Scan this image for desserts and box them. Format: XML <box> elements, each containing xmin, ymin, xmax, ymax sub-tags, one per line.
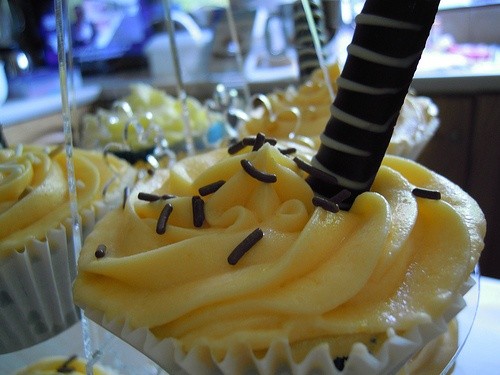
<box><xmin>79</xmin><ymin>85</ymin><xmax>210</xmax><ymax>151</ymax></box>
<box><xmin>0</xmin><ymin>143</ymin><xmax>127</xmax><ymax>261</ymax></box>
<box><xmin>72</xmin><ymin>134</ymin><xmax>486</xmax><ymax>374</ymax></box>
<box><xmin>239</xmin><ymin>58</ymin><xmax>431</xmax><ymax>143</ymax></box>
